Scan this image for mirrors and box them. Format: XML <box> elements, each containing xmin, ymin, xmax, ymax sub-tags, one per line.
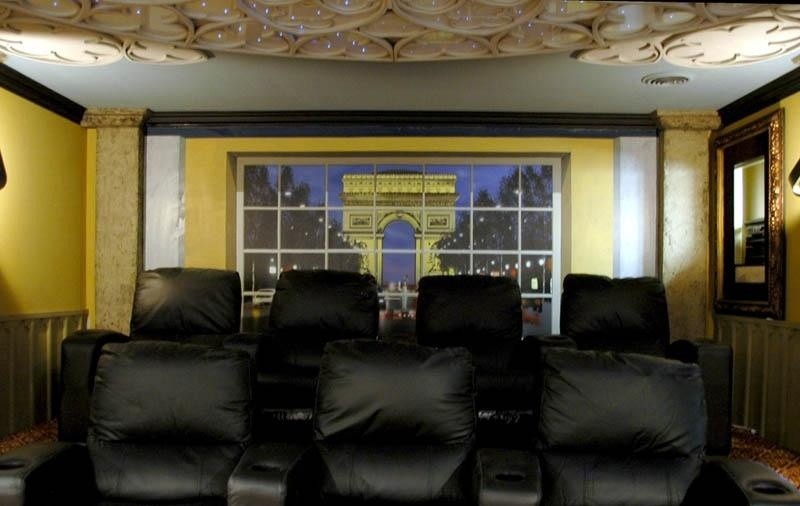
<box><xmin>709</xmin><ymin>107</ymin><xmax>786</xmax><ymax>322</ymax></box>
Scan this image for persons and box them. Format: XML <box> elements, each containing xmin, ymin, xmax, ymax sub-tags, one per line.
<box><xmin>522</xmin><ymin>298</ymin><xmax>542</xmax><ymax>313</ymax></box>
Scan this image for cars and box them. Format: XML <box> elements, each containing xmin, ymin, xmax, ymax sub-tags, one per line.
<box><xmin>253</xmin><ymin>288</ymin><xmax>276</xmax><ymax>307</ymax></box>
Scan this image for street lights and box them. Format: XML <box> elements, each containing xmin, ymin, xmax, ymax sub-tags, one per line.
<box><xmin>270</xmin><ymin>189</ymin><xmax>341</xmax><ymax>286</ymax></box>
<box><xmin>440</xmin><ymin>189</ymin><xmax>546</xmax><ymax>305</ymax></box>
